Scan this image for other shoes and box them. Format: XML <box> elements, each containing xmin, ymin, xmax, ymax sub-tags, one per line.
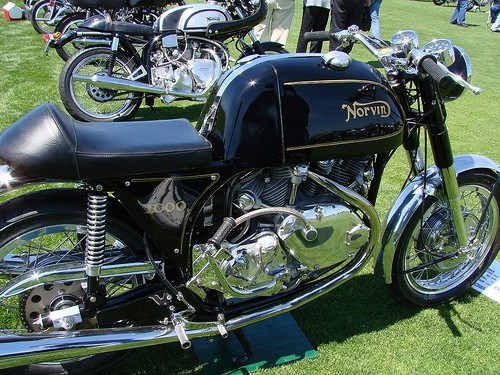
<box><xmin>459</xmin><ymin>23</ymin><xmax>469</xmax><ymax>27</ymax></box>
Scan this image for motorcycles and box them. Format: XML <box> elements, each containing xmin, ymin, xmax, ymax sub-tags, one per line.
<box><xmin>0</xmin><ymin>24</ymin><xmax>500</xmax><ymax>375</ymax></box>
<box><xmin>433</xmin><ymin>0</ymin><xmax>490</xmax><ymax>13</ymax></box>
<box><xmin>23</xmin><ymin>0</ymin><xmax>288</xmax><ymax>123</ymax></box>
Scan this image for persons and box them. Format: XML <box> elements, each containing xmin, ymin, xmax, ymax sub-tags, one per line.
<box><xmin>296</xmin><ymin>0</ymin><xmax>331</xmax><ymax>53</ymax></box>
<box><xmin>489</xmin><ymin>0</ymin><xmax>500</xmax><ymax>32</ymax></box>
<box><xmin>329</xmin><ymin>0</ymin><xmax>371</xmax><ymax>54</ymax></box>
<box><xmin>370</xmin><ymin>0</ymin><xmax>383</xmax><ymax>48</ymax></box>
<box><xmin>251</xmin><ymin>0</ymin><xmax>295</xmax><ymax>55</ymax></box>
<box><xmin>451</xmin><ymin>0</ymin><xmax>468</xmax><ymax>27</ymax></box>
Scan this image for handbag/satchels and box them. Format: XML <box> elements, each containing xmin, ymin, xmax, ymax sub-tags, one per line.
<box><xmin>361</xmin><ymin>5</ymin><xmax>372</xmax><ymax>32</ymax></box>
